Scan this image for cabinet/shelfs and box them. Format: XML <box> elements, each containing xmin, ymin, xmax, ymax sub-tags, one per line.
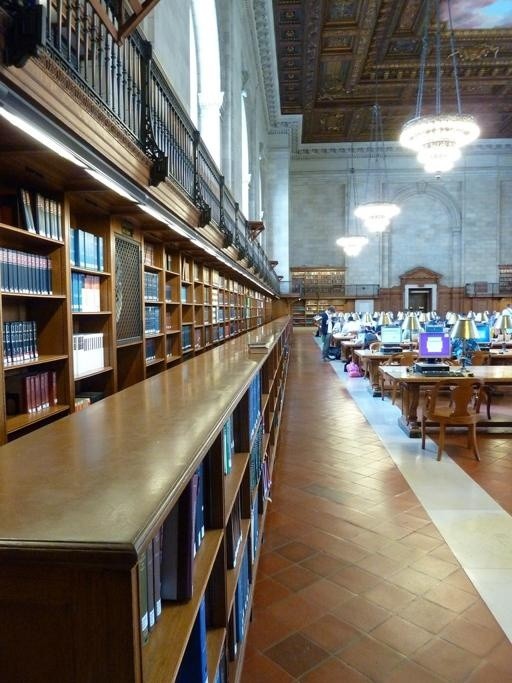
<box><xmin>1</xmin><ymin>131</ymin><xmax>292</xmax><ymax>683</ymax></box>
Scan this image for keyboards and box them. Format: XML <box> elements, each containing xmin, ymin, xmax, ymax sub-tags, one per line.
<box><xmin>383</xmin><ymin>352</ymin><xmax>397</xmax><ymax>354</ymax></box>
<box><xmin>422</xmin><ymin>371</ymin><xmax>465</xmax><ymax>377</ymax></box>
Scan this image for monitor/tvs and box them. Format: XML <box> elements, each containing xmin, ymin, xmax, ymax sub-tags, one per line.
<box><xmin>474</xmin><ymin>325</ymin><xmax>491</xmax><ymax>344</ymax></box>
<box><xmin>425</xmin><ymin>325</ymin><xmax>444</xmax><ymax>332</ymax></box>
<box><xmin>417</xmin><ymin>332</ymin><xmax>452</xmax><ymax>364</ymax></box>
<box><xmin>381</xmin><ymin>326</ymin><xmax>402</xmax><ymax>347</ymax></box>
<box><xmin>358</xmin><ymin>332</ymin><xmax>366</xmax><ymax>342</ymax></box>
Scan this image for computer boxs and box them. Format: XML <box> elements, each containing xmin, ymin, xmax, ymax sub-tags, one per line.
<box><xmin>480</xmin><ymin>347</ymin><xmax>490</xmax><ymax>352</ymax></box>
<box><xmin>380</xmin><ymin>346</ymin><xmax>402</xmax><ymax>353</ymax></box>
<box><xmin>416</xmin><ymin>362</ymin><xmax>449</xmax><ymax>374</ymax></box>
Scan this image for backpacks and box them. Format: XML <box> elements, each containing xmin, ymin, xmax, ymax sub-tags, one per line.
<box><xmin>345</xmin><ymin>361</ymin><xmax>362</xmax><ymax>377</ymax></box>
<box><xmin>327</xmin><ymin>346</ymin><xmax>340</xmax><ymax>360</ymax></box>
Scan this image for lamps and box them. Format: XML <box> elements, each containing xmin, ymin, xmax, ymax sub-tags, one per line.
<box><xmin>396</xmin><ymin>0</ymin><xmax>482</xmax><ymax>178</ymax></box>
<box><xmin>330</xmin><ymin>308</ymin><xmax>512</xmax><ymax>372</ymax></box>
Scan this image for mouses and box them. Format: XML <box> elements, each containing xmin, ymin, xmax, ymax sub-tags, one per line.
<box><xmin>499</xmin><ymin>351</ymin><xmax>503</xmax><ymax>354</ymax></box>
<box><xmin>468</xmin><ymin>372</ymin><xmax>474</xmax><ymax>376</ymax></box>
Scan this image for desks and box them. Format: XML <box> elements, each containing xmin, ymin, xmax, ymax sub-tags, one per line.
<box><xmin>313</xmin><ymin>316</ymin><xmax>511</xmax><ymax>438</ymax></box>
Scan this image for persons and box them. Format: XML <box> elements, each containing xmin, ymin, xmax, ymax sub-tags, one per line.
<box><xmin>318</xmin><ymin>305</ymin><xmax>336</xmax><ymax>362</ymax></box>
<box><xmin>506</xmin><ymin>303</ymin><xmax>512</xmax><ymax>315</ymax></box>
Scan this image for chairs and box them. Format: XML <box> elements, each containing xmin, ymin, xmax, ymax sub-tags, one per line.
<box><xmin>420</xmin><ymin>376</ymin><xmax>486</xmax><ymax>464</ymax></box>
